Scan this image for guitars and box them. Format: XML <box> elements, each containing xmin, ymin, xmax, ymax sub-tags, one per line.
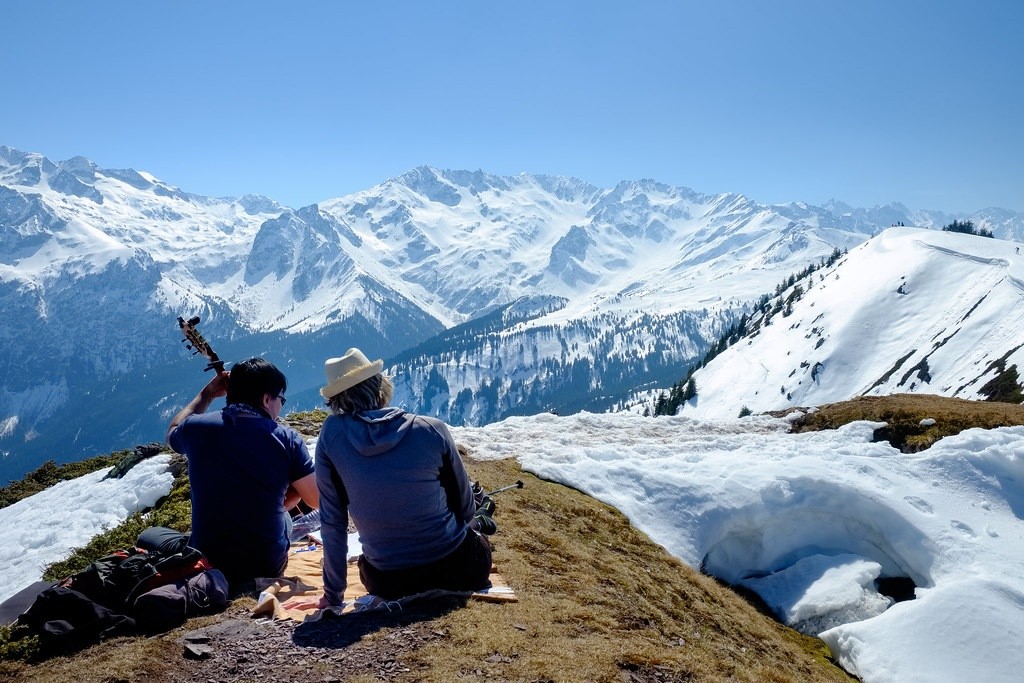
<box><xmin>175</xmin><ymin>315</ymin><xmax>301</xmax><ymax>510</ymax></box>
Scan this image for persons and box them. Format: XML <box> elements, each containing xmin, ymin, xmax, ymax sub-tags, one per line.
<box><xmin>281</xmin><ymin>347</ymin><xmax>494</xmax><ymax>612</ymax></box>
<box><xmin>136</xmin><ymin>356</ymin><xmax>322</xmax><ymax>598</ymax></box>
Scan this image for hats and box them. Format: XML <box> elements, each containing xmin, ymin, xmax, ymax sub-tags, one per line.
<box><xmin>320</xmin><ymin>347</ymin><xmax>384</xmax><ymax>401</ymax></box>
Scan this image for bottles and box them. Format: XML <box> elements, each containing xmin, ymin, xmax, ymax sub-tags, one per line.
<box><xmin>287</xmin><ymin>508</ymin><xmax>321</xmax><ymax>546</ymax></box>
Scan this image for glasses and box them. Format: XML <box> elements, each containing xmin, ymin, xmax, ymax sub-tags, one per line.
<box><xmin>272</xmin><ymin>395</ymin><xmax>286</xmax><ymax>406</ymax></box>
